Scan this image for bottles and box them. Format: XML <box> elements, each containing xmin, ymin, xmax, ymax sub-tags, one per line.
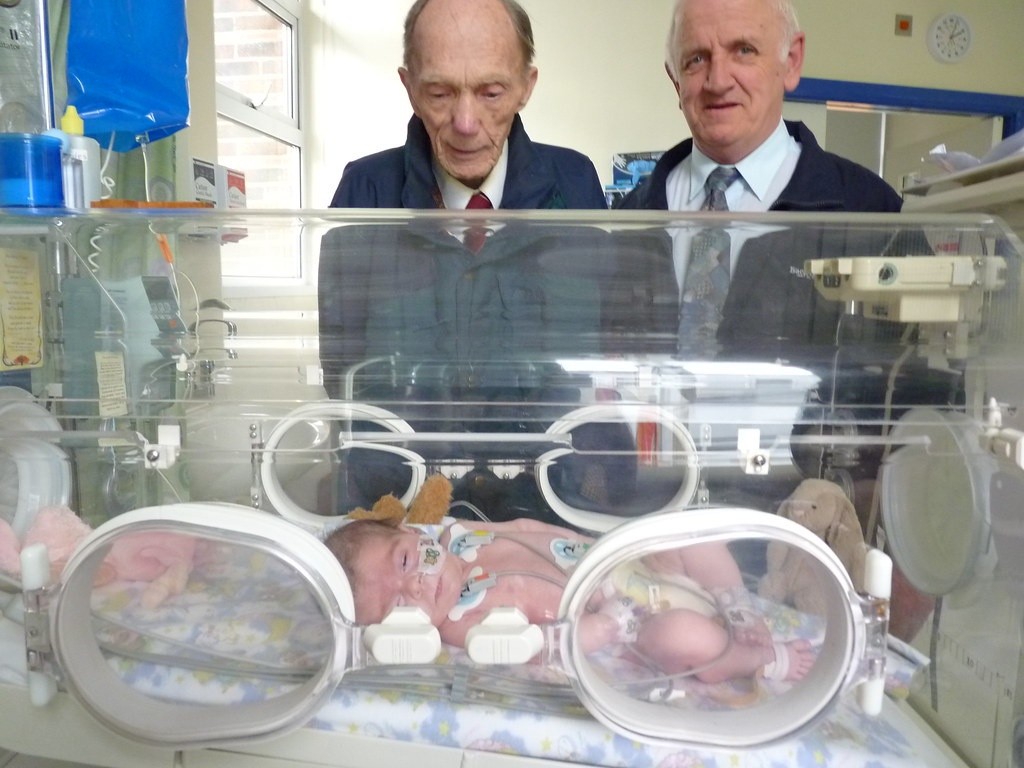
<box><xmin>61</xmin><ymin>106</ymin><xmax>100</xmax><ymax>208</ymax></box>
<box><xmin>39</xmin><ymin>129</ymin><xmax>84</xmax><ymax>208</ymax></box>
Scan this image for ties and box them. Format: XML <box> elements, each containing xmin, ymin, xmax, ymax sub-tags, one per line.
<box><xmin>677</xmin><ymin>166</ymin><xmax>742</xmax><ymax>360</ymax></box>
<box><xmin>463</xmin><ymin>192</ymin><xmax>493</xmax><ymax>253</ymax></box>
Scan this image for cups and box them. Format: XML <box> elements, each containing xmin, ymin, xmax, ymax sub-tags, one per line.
<box><xmin>0</xmin><ymin>133</ymin><xmax>64</xmax><ymax>208</ymax></box>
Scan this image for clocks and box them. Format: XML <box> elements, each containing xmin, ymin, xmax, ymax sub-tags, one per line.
<box><xmin>926</xmin><ymin>13</ymin><xmax>972</xmax><ymax>64</ymax></box>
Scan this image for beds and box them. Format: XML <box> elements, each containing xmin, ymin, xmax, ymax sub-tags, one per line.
<box><xmin>0</xmin><ymin>549</ymin><xmax>956</xmax><ymax>768</ymax></box>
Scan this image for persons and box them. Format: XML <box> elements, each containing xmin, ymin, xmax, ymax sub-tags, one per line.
<box><xmin>320</xmin><ymin>506</ymin><xmax>817</xmax><ymax>687</ymax></box>
<box><xmin>314</xmin><ymin>0</ymin><xmax>635</xmax><ymax>519</ymax></box>
<box><xmin>602</xmin><ymin>1</ymin><xmax>968</xmax><ymax>641</ymax></box>
